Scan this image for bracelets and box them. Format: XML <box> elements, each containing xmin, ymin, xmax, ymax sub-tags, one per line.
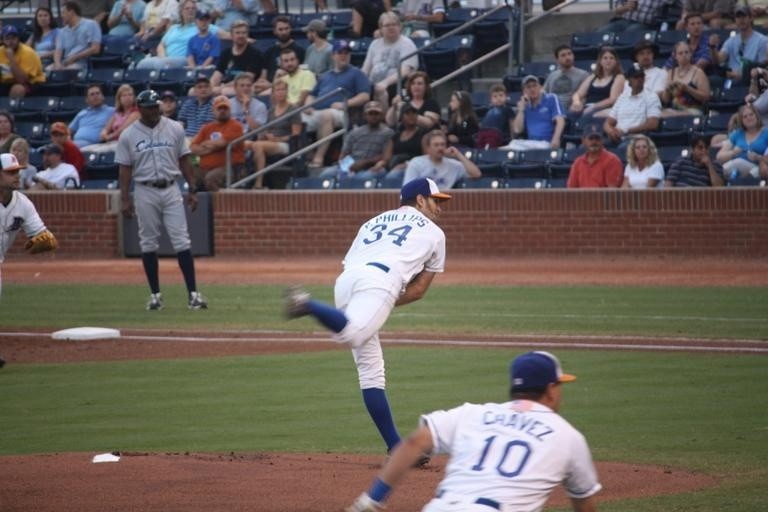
<box><xmin>189</xmin><ymin>186</ymin><xmax>198</xmax><ymax>193</ymax></box>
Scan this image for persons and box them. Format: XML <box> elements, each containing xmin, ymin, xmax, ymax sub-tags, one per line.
<box><xmin>0</xmin><ymin>0</ymin><xmax>767</xmax><ymax>190</ymax></box>
<box><xmin>0</xmin><ymin>153</ymin><xmax>59</xmax><ymax>369</ymax></box>
<box><xmin>114</xmin><ymin>89</ymin><xmax>209</xmax><ymax>311</ymax></box>
<box><xmin>342</xmin><ymin>350</ymin><xmax>602</xmax><ymax>512</ymax></box>
<box><xmin>284</xmin><ymin>176</ymin><xmax>452</xmax><ymax>468</ymax></box>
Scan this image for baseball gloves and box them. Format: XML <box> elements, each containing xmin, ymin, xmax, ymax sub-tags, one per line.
<box><xmin>24</xmin><ymin>231</ymin><xmax>59</xmax><ymax>253</ymax></box>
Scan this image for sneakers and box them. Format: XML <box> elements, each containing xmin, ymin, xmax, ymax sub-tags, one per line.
<box><xmin>188</xmin><ymin>292</ymin><xmax>208</xmax><ymax>309</ymax></box>
<box><xmin>146</xmin><ymin>293</ymin><xmax>162</xmax><ymax>310</ymax></box>
<box><xmin>285</xmin><ymin>293</ymin><xmax>309</xmax><ymax>319</ymax></box>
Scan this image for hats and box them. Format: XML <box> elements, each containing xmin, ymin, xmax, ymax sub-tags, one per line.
<box><xmin>399</xmin><ymin>177</ymin><xmax>452</xmax><ymax>203</ymax></box>
<box><xmin>522</xmin><ymin>40</ymin><xmax>660</xmax><ymax>138</ymax></box>
<box><xmin>510</xmin><ymin>351</ymin><xmax>576</xmax><ymax>390</ymax></box>
<box><xmin>732</xmin><ymin>6</ymin><xmax>750</xmax><ymax>19</ymax></box>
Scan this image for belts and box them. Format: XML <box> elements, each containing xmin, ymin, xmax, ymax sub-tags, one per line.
<box><xmin>140</xmin><ymin>180</ymin><xmax>174</xmax><ymax>188</ymax></box>
